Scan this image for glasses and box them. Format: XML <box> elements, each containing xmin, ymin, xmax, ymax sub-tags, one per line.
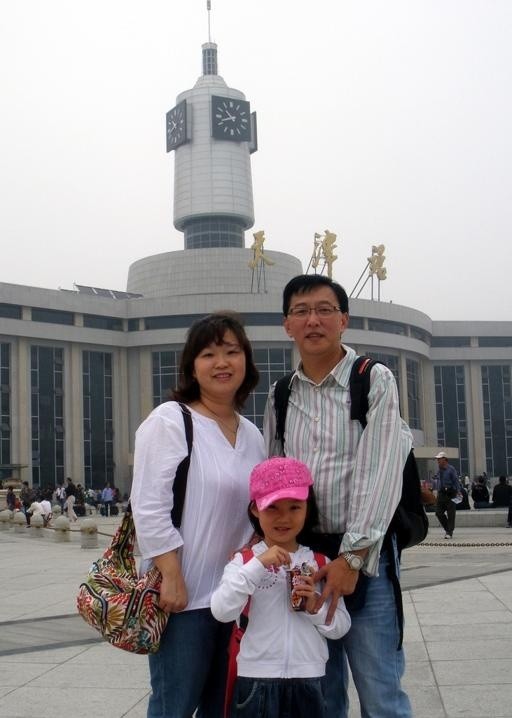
<box><xmin>285</xmin><ymin>305</ymin><xmax>345</xmax><ymax>321</ymax></box>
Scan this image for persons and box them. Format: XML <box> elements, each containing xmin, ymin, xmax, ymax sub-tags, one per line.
<box><xmin>420</xmin><ymin>471</ymin><xmax>512</xmax><ymax>528</ymax></box>
<box><xmin>6</xmin><ymin>474</ymin><xmax>118</xmax><ymax>528</ymax></box>
<box><xmin>261</xmin><ymin>275</ymin><xmax>423</xmax><ymax>718</ymax></box>
<box><xmin>434</xmin><ymin>452</ymin><xmax>462</xmax><ymax>539</ymax></box>
<box><xmin>123</xmin><ymin>314</ymin><xmax>265</xmax><ymax>718</ymax></box>
<box><xmin>211</xmin><ymin>453</ymin><xmax>357</xmax><ymax>718</ymax></box>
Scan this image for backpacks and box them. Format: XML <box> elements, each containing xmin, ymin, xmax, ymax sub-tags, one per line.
<box><xmin>274</xmin><ymin>355</ymin><xmax>429</xmax><ymax>551</ymax></box>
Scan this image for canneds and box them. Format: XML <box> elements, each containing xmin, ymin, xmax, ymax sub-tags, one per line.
<box><xmin>286</xmin><ymin>569</ymin><xmax>307</xmax><ymax>613</ymax></box>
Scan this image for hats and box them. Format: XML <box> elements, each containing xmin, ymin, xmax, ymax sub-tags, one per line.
<box><xmin>249</xmin><ymin>455</ymin><xmax>314</xmax><ymax>512</ymax></box>
<box><xmin>434</xmin><ymin>452</ymin><xmax>447</xmax><ymax>458</ymax></box>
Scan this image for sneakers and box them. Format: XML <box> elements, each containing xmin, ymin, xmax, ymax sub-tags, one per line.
<box><xmin>444</xmin><ymin>534</ymin><xmax>452</xmax><ymax>539</ymax></box>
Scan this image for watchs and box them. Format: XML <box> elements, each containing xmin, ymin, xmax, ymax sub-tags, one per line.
<box><xmin>341</xmin><ymin>552</ymin><xmax>363</xmax><ymax>571</ymax></box>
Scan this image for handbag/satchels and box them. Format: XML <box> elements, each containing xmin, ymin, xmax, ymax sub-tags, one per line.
<box><xmin>77</xmin><ymin>514</ymin><xmax>179</xmax><ymax>654</ymax></box>
